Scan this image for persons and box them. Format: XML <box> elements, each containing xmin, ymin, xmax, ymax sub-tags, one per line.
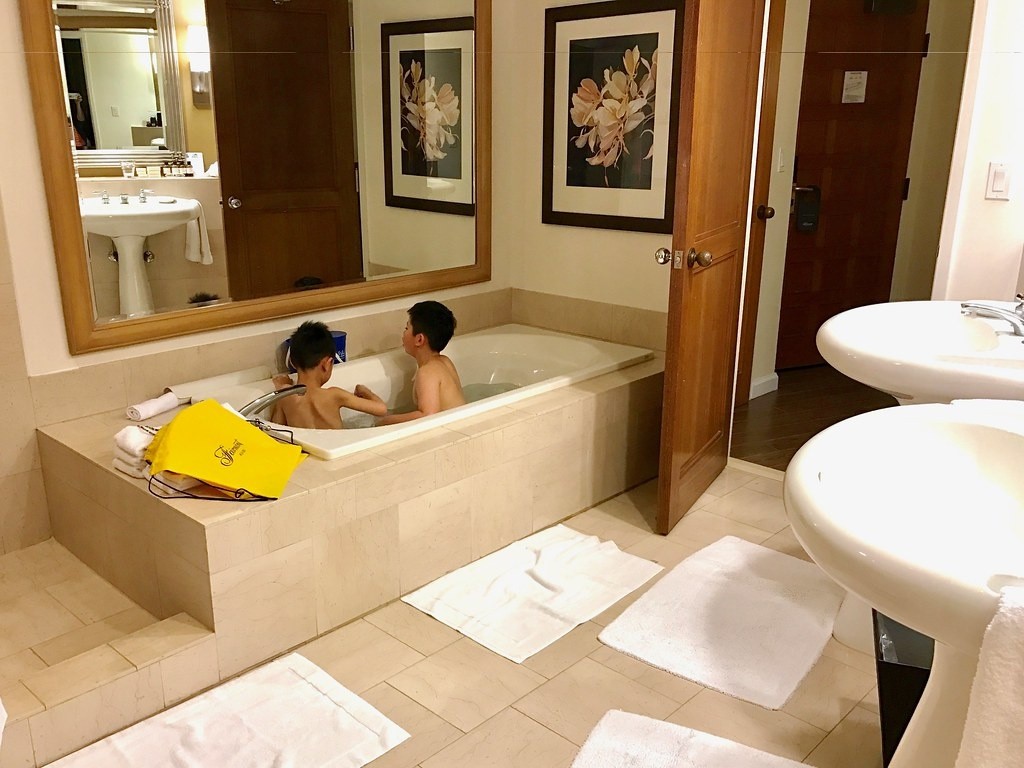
<box><xmin>378</xmin><ymin>301</ymin><xmax>464</xmax><ymax>422</ymax></box>
<box><xmin>271</xmin><ymin>320</ymin><xmax>387</xmax><ymax>429</ymax></box>
<box><xmin>187</xmin><ymin>293</ymin><xmax>220</xmax><ymax>307</ymax></box>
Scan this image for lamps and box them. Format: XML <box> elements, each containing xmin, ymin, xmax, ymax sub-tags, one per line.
<box><xmin>187</xmin><ymin>25</ymin><xmax>211</xmax><ymax>94</ymax></box>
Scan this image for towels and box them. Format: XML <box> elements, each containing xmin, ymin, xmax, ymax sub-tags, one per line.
<box><xmin>125</xmin><ymin>391</ymin><xmax>179</xmax><ymax>422</ymax></box>
<box><xmin>112</xmin><ymin>425</ymin><xmax>203</xmax><ymax>495</ymax></box>
<box><xmin>568</xmin><ymin>708</ymin><xmax>821</xmax><ymax>768</ymax></box>
<box><xmin>400</xmin><ymin>523</ymin><xmax>665</xmax><ymax>665</ymax></box>
<box><xmin>951</xmin><ymin>583</ymin><xmax>1024</xmax><ymax>768</ymax></box>
<box><xmin>596</xmin><ymin>534</ymin><xmax>848</xmax><ymax>710</ymax></box>
<box><xmin>185</xmin><ymin>199</ymin><xmax>213</xmax><ymax>266</ymax></box>
<box><xmin>40</xmin><ymin>652</ymin><xmax>412</xmax><ymax>768</ymax></box>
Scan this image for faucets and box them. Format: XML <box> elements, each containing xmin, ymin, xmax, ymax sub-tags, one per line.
<box><xmin>119</xmin><ymin>192</ymin><xmax>129</xmax><ymax>205</ymax></box>
<box><xmin>237</xmin><ymin>383</ymin><xmax>308</xmax><ymax>421</ymax></box>
<box><xmin>960</xmin><ymin>301</ymin><xmax>1024</xmax><ymax>336</ymax></box>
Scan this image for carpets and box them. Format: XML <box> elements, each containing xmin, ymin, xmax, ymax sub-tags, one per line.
<box><xmin>39</xmin><ymin>651</ymin><xmax>411</xmax><ymax>768</ymax></box>
<box><xmin>597</xmin><ymin>535</ymin><xmax>846</xmax><ymax>711</ymax></box>
<box><xmin>398</xmin><ymin>522</ymin><xmax>666</xmax><ymax>664</ymax></box>
<box><xmin>570</xmin><ymin>710</ymin><xmax>818</xmax><ymax>768</ymax></box>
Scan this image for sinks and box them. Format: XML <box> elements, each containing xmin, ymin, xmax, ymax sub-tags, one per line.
<box><xmin>816</xmin><ymin>299</ymin><xmax>1024</xmax><ymax>400</ymax></box>
<box><xmin>782</xmin><ymin>397</ymin><xmax>1024</xmax><ymax>656</ymax></box>
<box><xmin>80</xmin><ymin>195</ymin><xmax>201</xmax><ymax>236</ymax></box>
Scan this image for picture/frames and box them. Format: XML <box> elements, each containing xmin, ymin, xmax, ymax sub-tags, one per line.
<box><xmin>541</xmin><ymin>0</ymin><xmax>688</xmax><ymax>236</ymax></box>
<box><xmin>379</xmin><ymin>14</ymin><xmax>478</xmax><ymax>217</ymax></box>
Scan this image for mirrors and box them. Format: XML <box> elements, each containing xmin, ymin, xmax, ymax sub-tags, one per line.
<box><xmin>16</xmin><ymin>0</ymin><xmax>493</xmax><ymax>356</ymax></box>
<box><xmin>52</xmin><ymin>0</ymin><xmax>187</xmax><ymax>169</ymax></box>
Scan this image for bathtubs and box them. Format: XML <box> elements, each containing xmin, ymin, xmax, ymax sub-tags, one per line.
<box><xmin>38</xmin><ymin>320</ymin><xmax>666</xmax><ymax>682</ymax></box>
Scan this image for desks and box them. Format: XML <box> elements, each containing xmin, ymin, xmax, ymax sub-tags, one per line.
<box><xmin>131</xmin><ymin>127</ymin><xmax>166</xmax><ymax>147</ymax></box>
